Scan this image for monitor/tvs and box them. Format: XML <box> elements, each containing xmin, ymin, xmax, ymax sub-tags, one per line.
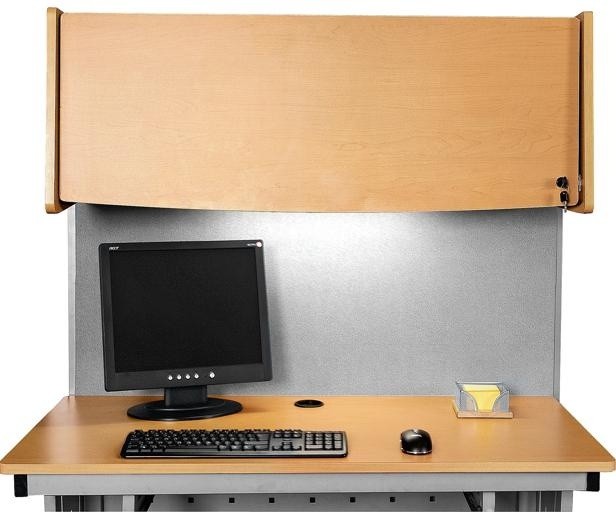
<box><xmin>99</xmin><ymin>239</ymin><xmax>272</xmax><ymax>422</ymax></box>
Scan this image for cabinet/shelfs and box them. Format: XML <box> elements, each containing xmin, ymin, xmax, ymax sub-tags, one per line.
<box><xmin>45</xmin><ymin>7</ymin><xmax>593</xmax><ymax>215</ymax></box>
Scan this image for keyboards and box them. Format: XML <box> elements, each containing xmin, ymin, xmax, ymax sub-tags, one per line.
<box><xmin>119</xmin><ymin>429</ymin><xmax>347</xmax><ymax>458</ymax></box>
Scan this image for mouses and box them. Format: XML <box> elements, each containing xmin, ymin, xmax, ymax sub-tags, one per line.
<box><xmin>400</xmin><ymin>429</ymin><xmax>432</xmax><ymax>455</ymax></box>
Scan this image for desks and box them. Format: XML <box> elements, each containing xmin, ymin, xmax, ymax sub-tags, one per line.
<box><xmin>0</xmin><ymin>396</ymin><xmax>615</xmax><ymax>512</ymax></box>
